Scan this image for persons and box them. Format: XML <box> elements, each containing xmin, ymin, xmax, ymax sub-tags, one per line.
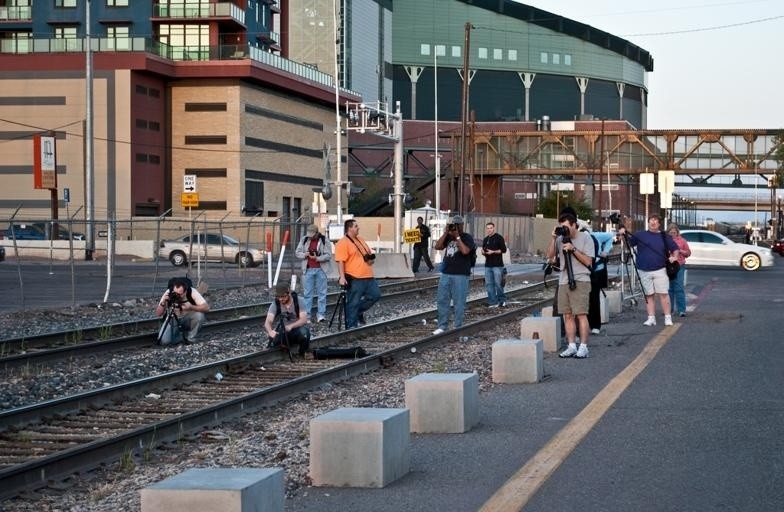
<box><xmin>155</xmin><ymin>281</ymin><xmax>210</xmax><ymax>344</ymax></box>
<box><xmin>335</xmin><ymin>219</ymin><xmax>381</xmax><ymax>328</ymax></box>
<box><xmin>546</xmin><ymin>213</ymin><xmax>595</xmax><ymax>359</ymax></box>
<box><xmin>295</xmin><ymin>225</ymin><xmax>331</xmax><ymax>324</ymax></box>
<box><xmin>265</xmin><ymin>282</ymin><xmax>311</xmax><ymax>358</ymax></box>
<box><xmin>412</xmin><ymin>216</ymin><xmax>434</xmax><ymax>272</ymax></box>
<box><xmin>432</xmin><ymin>214</ymin><xmax>474</xmax><ymax>336</ymax></box>
<box><xmin>619</xmin><ymin>213</ymin><xmax>680</xmax><ymax>327</ymax></box>
<box><xmin>744</xmin><ymin>231</ymin><xmax>750</xmax><ymax>244</ymax></box>
<box><xmin>481</xmin><ymin>223</ymin><xmax>507</xmax><ymax>308</ymax></box>
<box><xmin>576</xmin><ymin>220</ymin><xmax>614</xmax><ymax>335</ymax></box>
<box><xmin>662</xmin><ymin>222</ymin><xmax>692</xmax><ymax>318</ymax></box>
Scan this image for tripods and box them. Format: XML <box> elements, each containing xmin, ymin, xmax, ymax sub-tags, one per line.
<box><xmin>617</xmin><ymin>223</ymin><xmax>649</xmax><ymax>306</ymax></box>
<box><xmin>156</xmin><ymin>307</ymin><xmax>191</xmax><ymax>347</ymax></box>
<box><xmin>328</xmin><ymin>290</ymin><xmax>351</xmax><ymax>332</ymax></box>
<box><xmin>265</xmin><ymin>315</ymin><xmax>297</xmax><ymax>364</ymax></box>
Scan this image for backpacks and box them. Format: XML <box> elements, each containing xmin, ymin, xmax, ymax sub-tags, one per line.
<box><xmin>666</xmin><ymin>252</ymin><xmax>680</xmax><ymax>281</ymax></box>
<box><xmin>167</xmin><ymin>277</ymin><xmax>193</xmax><ymax>291</ymax></box>
<box><xmin>469</xmin><ymin>242</ymin><xmax>477</xmax><ymax>268</ymax></box>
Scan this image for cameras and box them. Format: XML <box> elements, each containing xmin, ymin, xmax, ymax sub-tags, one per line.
<box><xmin>308</xmin><ymin>247</ymin><xmax>321</xmax><ymax>256</ymax></box>
<box><xmin>610</xmin><ymin>213</ymin><xmax>621</xmax><ymax>224</ymax></box>
<box><xmin>555</xmin><ymin>225</ymin><xmax>570</xmax><ymax>237</ymax></box>
<box><xmin>167</xmin><ymin>292</ymin><xmax>180</xmax><ymax>303</ymax></box>
<box><xmin>338</xmin><ymin>273</ymin><xmax>354</xmax><ymax>290</ymax></box>
<box><xmin>449</xmin><ymin>223</ymin><xmax>459</xmax><ymax>231</ymax></box>
<box><xmin>363</xmin><ymin>254</ymin><xmax>376</xmax><ymax>261</ymax></box>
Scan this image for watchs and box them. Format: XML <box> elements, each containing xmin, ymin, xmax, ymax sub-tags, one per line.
<box><xmin>571</xmin><ymin>246</ymin><xmax>577</xmax><ymax>253</ymax></box>
<box><xmin>456</xmin><ymin>237</ymin><xmax>461</xmax><ymax>241</ymax></box>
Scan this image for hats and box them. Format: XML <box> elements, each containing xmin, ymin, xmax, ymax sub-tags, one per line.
<box><xmin>272</xmin><ymin>282</ymin><xmax>290</xmax><ymax>296</ymax></box>
<box><xmin>305</xmin><ymin>224</ymin><xmax>318</xmax><ymax>238</ymax></box>
<box><xmin>453</xmin><ymin>216</ymin><xmax>465</xmax><ymax>224</ymax></box>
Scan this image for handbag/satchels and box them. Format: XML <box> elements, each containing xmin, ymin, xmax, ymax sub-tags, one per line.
<box><xmin>660</xmin><ymin>230</ymin><xmax>681</xmax><ymax>278</ymax></box>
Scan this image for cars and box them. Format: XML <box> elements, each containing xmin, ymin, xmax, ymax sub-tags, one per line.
<box><xmin>6</xmin><ymin>222</ymin><xmax>85</xmax><ymax>241</ymax></box>
<box><xmin>680</xmin><ymin>230</ymin><xmax>773</xmax><ymax>271</ymax></box>
<box><xmin>153</xmin><ymin>233</ymin><xmax>274</xmax><ymax>266</ymax></box>
<box><xmin>772</xmin><ymin>239</ymin><xmax>784</xmax><ymax>257</ymax></box>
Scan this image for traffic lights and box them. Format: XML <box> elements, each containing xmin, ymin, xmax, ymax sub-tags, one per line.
<box><xmin>64</xmin><ymin>188</ymin><xmax>69</xmax><ymax>202</ymax></box>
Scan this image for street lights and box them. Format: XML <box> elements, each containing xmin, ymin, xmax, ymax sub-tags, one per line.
<box><xmin>777</xmin><ymin>196</ymin><xmax>782</xmax><ymax>239</ymax></box>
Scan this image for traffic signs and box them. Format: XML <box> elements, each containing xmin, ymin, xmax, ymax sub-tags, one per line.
<box><xmin>180</xmin><ymin>176</ymin><xmax>198</xmax><ymax>207</ymax></box>
<box><xmin>34</xmin><ymin>136</ymin><xmax>57</xmax><ymax>189</ymax></box>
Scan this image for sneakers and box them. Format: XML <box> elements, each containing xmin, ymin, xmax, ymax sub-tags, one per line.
<box><xmin>187</xmin><ymin>339</ymin><xmax>199</xmax><ymax>345</ymax></box>
<box><xmin>664</xmin><ymin>314</ymin><xmax>674</xmax><ymax>326</ymax></box>
<box><xmin>307</xmin><ymin>319</ymin><xmax>312</xmax><ymax>324</ymax></box>
<box><xmin>428</xmin><ymin>267</ymin><xmax>435</xmax><ymax>272</ymax></box>
<box><xmin>489</xmin><ymin>301</ymin><xmax>507</xmax><ymax>308</ymax></box>
<box><xmin>432</xmin><ymin>329</ymin><xmax>450</xmax><ymax>335</ymax></box>
<box><xmin>593</xmin><ymin>328</ymin><xmax>601</xmax><ymax>335</ymax></box>
<box><xmin>560</xmin><ymin>343</ymin><xmax>579</xmax><ymax>358</ymax></box>
<box><xmin>577</xmin><ymin>343</ymin><xmax>590</xmax><ymax>359</ymax></box>
<box><xmin>349</xmin><ymin>314</ymin><xmax>366</xmax><ymax>330</ymax></box>
<box><xmin>680</xmin><ymin>308</ymin><xmax>687</xmax><ymax>317</ymax></box>
<box><xmin>644</xmin><ymin>316</ymin><xmax>658</xmax><ymax>326</ymax></box>
<box><xmin>299</xmin><ymin>347</ymin><xmax>314</xmax><ymax>361</ymax></box>
<box><xmin>317</xmin><ymin>314</ymin><xmax>327</xmax><ymax>322</ymax></box>
